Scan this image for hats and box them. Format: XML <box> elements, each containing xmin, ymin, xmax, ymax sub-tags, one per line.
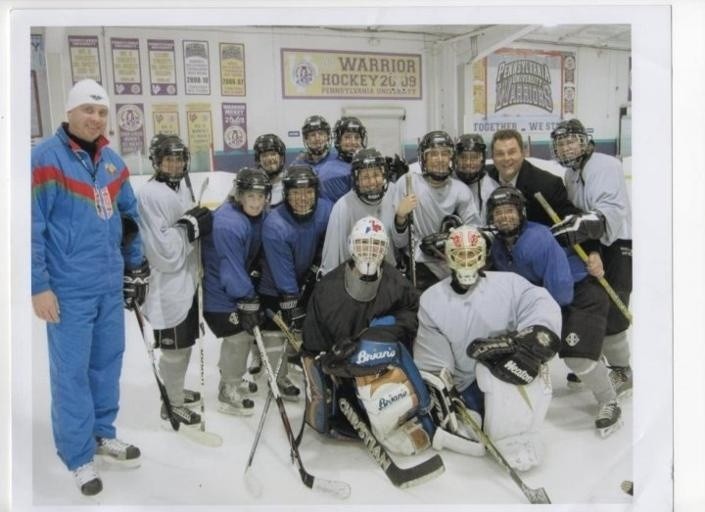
<box><xmin>64</xmin><ymin>76</ymin><xmax>111</xmax><ymax>114</ymax></box>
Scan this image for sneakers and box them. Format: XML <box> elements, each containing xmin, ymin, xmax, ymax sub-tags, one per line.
<box><xmin>234</xmin><ymin>376</ymin><xmax>259</xmax><ymax>395</ymax></box>
<box><xmin>247</xmin><ymin>344</ymin><xmax>265</xmax><ymax>374</ymax></box>
<box><xmin>566</xmin><ymin>372</ymin><xmax>582</xmax><ymax>383</ymax></box>
<box><xmin>267</xmin><ymin>371</ymin><xmax>301</xmax><ymax>397</ymax></box>
<box><xmin>610</xmin><ymin>364</ymin><xmax>635</xmax><ymax>396</ymax></box>
<box><xmin>158</xmin><ymin>400</ymin><xmax>203</xmax><ymax>426</ymax></box>
<box><xmin>595</xmin><ymin>398</ymin><xmax>623</xmax><ymax>429</ymax></box>
<box><xmin>217</xmin><ymin>388</ymin><xmax>255</xmax><ymax>410</ymax></box>
<box><xmin>71</xmin><ymin>459</ymin><xmax>105</xmax><ymax>497</ymax></box>
<box><xmin>95</xmin><ymin>433</ymin><xmax>142</xmax><ymax>462</ymax></box>
<box><xmin>181</xmin><ymin>387</ymin><xmax>201</xmax><ymax>404</ymax></box>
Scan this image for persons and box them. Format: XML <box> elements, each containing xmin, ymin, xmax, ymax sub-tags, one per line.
<box><xmin>31</xmin><ymin>79</ymin><xmax>150</xmax><ymax>496</ymax></box>
<box><xmin>128</xmin><ymin>136</ymin><xmax>213</xmax><ymax>427</ymax></box>
<box><xmin>200</xmin><ymin>116</ymin><xmax>631</xmax><ymax>476</ymax></box>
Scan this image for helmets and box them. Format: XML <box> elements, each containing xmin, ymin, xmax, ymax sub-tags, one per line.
<box><xmin>334</xmin><ymin>114</ymin><xmax>368</xmax><ymax>142</ymax></box>
<box><xmin>485</xmin><ymin>185</ymin><xmax>529</xmax><ymax>215</ymax></box>
<box><xmin>147</xmin><ymin>132</ymin><xmax>191</xmax><ymax>168</ymax></box>
<box><xmin>453</xmin><ymin>131</ymin><xmax>488</xmax><ymax>159</ymax></box>
<box><xmin>233</xmin><ymin>164</ymin><xmax>273</xmax><ymax>194</ymax></box>
<box><xmin>347</xmin><ymin>216</ymin><xmax>392</xmax><ymax>248</ymax></box>
<box><xmin>444</xmin><ymin>223</ymin><xmax>489</xmax><ymax>260</ymax></box>
<box><xmin>549</xmin><ymin>118</ymin><xmax>589</xmax><ymax>146</ymax></box>
<box><xmin>252</xmin><ymin>133</ymin><xmax>287</xmax><ymax>157</ymax></box>
<box><xmin>418</xmin><ymin>129</ymin><xmax>457</xmax><ymax>158</ymax></box>
<box><xmin>348</xmin><ymin>147</ymin><xmax>388</xmax><ymax>172</ymax></box>
<box><xmin>280</xmin><ymin>164</ymin><xmax>322</xmax><ymax>196</ymax></box>
<box><xmin>300</xmin><ymin>115</ymin><xmax>332</xmax><ymax>138</ymax></box>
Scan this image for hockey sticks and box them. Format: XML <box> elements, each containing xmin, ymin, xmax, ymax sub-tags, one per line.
<box><xmin>456</xmin><ymin>403</ymin><xmax>551</xmax><ymax>504</ymax></box>
<box><xmin>197</xmin><ymin>177</ymin><xmax>209</xmax><ymax>431</ymax></box>
<box><xmin>130</xmin><ymin>299</ymin><xmax>221</xmax><ymax>447</ymax></box>
<box><xmin>243</xmin><ymin>308</ymin><xmax>446</xmax><ymax>495</ymax></box>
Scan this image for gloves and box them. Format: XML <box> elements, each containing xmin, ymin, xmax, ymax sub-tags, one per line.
<box><xmin>488</xmin><ymin>322</ymin><xmax>562</xmax><ymax>386</ymax></box>
<box><xmin>420</xmin><ymin>228</ymin><xmax>450</xmax><ymax>263</ymax></box>
<box><xmin>120</xmin><ymin>257</ymin><xmax>154</xmax><ymax>312</ymax></box>
<box><xmin>275</xmin><ymin>290</ymin><xmax>307</xmax><ymax>334</ymax></box>
<box><xmin>547</xmin><ymin>208</ymin><xmax>610</xmax><ymax>250</ymax></box>
<box><xmin>174</xmin><ymin>204</ymin><xmax>215</xmax><ymax>243</ymax></box>
<box><xmin>232</xmin><ymin>295</ymin><xmax>270</xmax><ymax>338</ymax></box>
